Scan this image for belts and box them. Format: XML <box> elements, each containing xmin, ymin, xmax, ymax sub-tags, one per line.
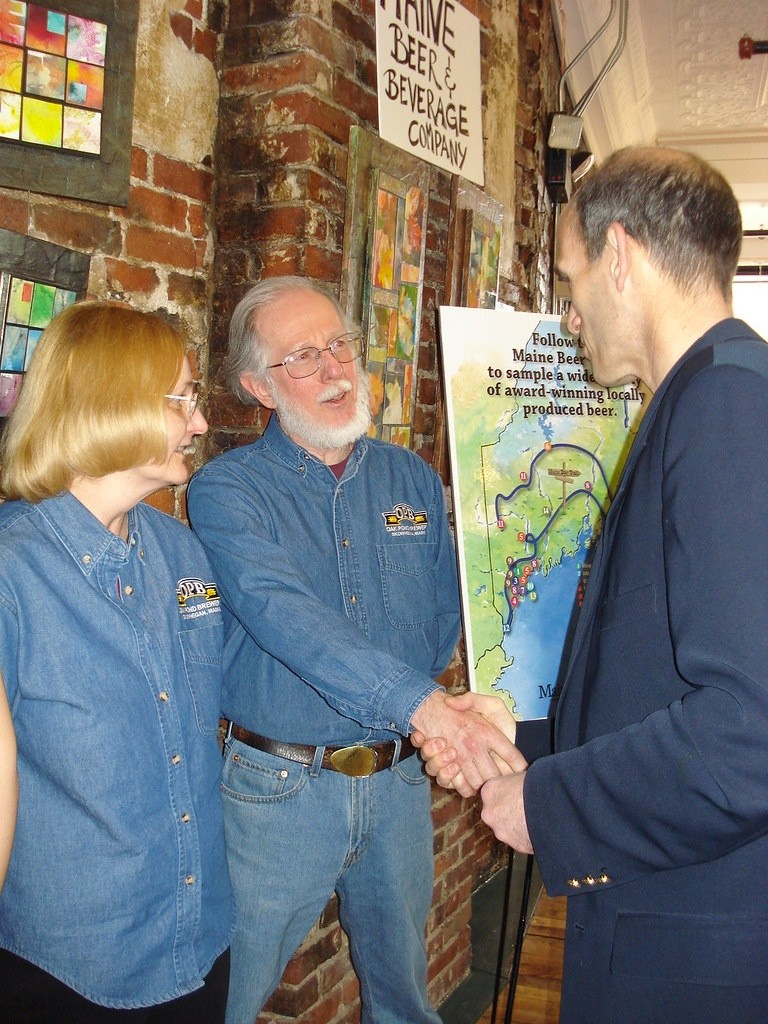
<box><xmin>229</xmin><ymin>721</ymin><xmax>417</xmax><ymax>777</ymax></box>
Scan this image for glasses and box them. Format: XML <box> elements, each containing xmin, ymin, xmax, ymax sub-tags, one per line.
<box><xmin>165</xmin><ymin>380</ymin><xmax>200</xmax><ymax>415</ymax></box>
<box><xmin>267</xmin><ymin>333</ymin><xmax>367</xmax><ymax>378</ymax></box>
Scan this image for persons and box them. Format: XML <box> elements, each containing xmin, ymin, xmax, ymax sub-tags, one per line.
<box><xmin>0</xmin><ymin>302</ymin><xmax>231</xmax><ymax>1022</ymax></box>
<box><xmin>406</xmin><ymin>139</ymin><xmax>768</xmax><ymax>1024</ymax></box>
<box><xmin>2</xmin><ymin>674</ymin><xmax>21</xmax><ymax>893</ymax></box>
<box><xmin>173</xmin><ymin>271</ymin><xmax>528</xmax><ymax>1019</ymax></box>
